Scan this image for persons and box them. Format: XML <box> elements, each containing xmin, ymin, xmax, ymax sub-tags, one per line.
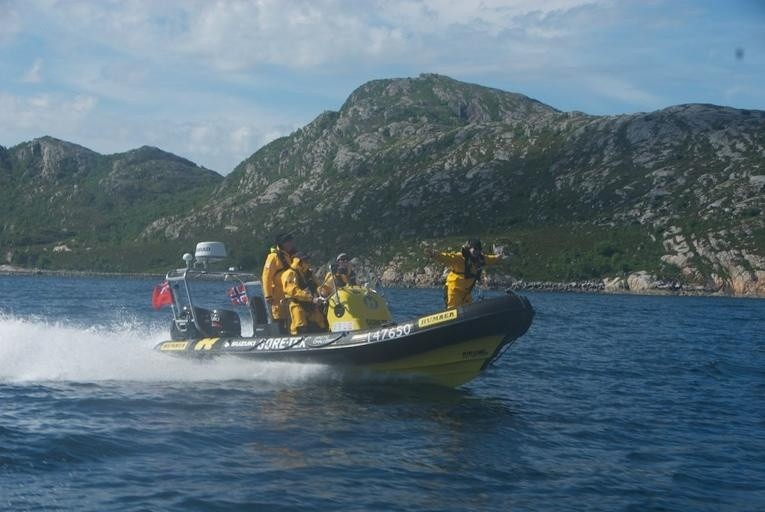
<box><xmin>423</xmin><ymin>238</ymin><xmax>504</xmax><ymax>310</ymax></box>
<box><xmin>261</xmin><ymin>230</ymin><xmax>357</xmax><ymax>336</ymax></box>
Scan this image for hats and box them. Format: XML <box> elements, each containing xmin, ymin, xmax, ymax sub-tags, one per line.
<box><xmin>274</xmin><ymin>232</ymin><xmax>295</xmax><ymax>243</ymax></box>
<box><xmin>293</xmin><ymin>250</ymin><xmax>311</xmax><ymax>260</ymax></box>
<box><xmin>335</xmin><ymin>249</ymin><xmax>347</xmax><ymax>260</ymax></box>
<box><xmin>469</xmin><ymin>239</ymin><xmax>482</xmax><ymax>249</ymax></box>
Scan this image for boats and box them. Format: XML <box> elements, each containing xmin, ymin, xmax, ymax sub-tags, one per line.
<box><xmin>154</xmin><ymin>241</ymin><xmax>536</xmax><ymax>390</ymax></box>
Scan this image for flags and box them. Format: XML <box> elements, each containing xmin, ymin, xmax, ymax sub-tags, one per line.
<box><xmin>152</xmin><ymin>282</ymin><xmax>175</xmax><ymax>312</ymax></box>
<box><xmin>227</xmin><ymin>284</ymin><xmax>248</xmax><ymax>305</ymax></box>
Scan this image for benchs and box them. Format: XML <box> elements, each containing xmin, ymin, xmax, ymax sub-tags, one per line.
<box><xmin>248</xmin><ymin>296</ymin><xmax>287</xmax><ymax>337</ymax></box>
<box><xmin>169</xmin><ymin>298</ymin><xmax>242</xmax><ymax>340</ymax></box>
